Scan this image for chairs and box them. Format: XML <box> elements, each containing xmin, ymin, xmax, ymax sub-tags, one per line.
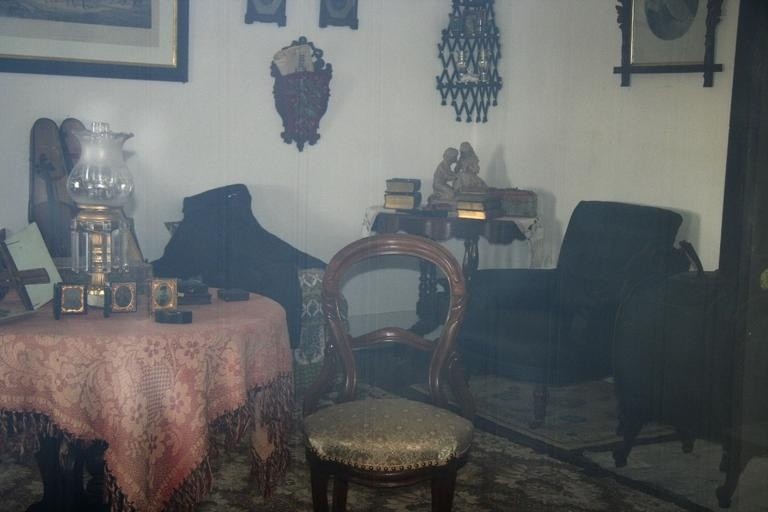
<box><xmin>298</xmin><ymin>232</ymin><xmax>476</xmax><ymax>512</ymax></box>
<box><xmin>455</xmin><ymin>200</ymin><xmax>683</xmax><ymax>422</ymax></box>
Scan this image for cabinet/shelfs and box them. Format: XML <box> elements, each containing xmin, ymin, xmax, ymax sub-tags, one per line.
<box><xmin>435</xmin><ymin>0</ymin><xmax>503</xmax><ymax>123</ymax></box>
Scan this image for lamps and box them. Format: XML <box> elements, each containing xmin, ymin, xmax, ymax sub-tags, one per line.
<box><xmin>66</xmin><ymin>121</ymin><xmax>135</xmax><ymax>307</ymax></box>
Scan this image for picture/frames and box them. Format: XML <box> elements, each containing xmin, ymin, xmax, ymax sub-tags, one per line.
<box><xmin>0</xmin><ymin>0</ymin><xmax>189</xmax><ymax>82</ymax></box>
<box><xmin>613</xmin><ymin>0</ymin><xmax>723</xmax><ymax>88</ymax></box>
<box><xmin>319</xmin><ymin>0</ymin><xmax>359</xmax><ymax>30</ymax></box>
<box><xmin>244</xmin><ymin>0</ymin><xmax>286</xmax><ymax>27</ymax></box>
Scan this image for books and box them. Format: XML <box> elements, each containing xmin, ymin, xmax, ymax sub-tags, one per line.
<box><xmin>456</xmin><ymin>207</ymin><xmax>498</xmax><ymax>221</ymax></box>
<box><xmin>0</xmin><ymin>222</ymin><xmax>63</xmax><ymax>311</ymax></box>
<box><xmin>453</xmin><ymin>194</ymin><xmax>497</xmax><ymax>211</ymax></box>
<box><xmin>382</xmin><ymin>177</ymin><xmax>419</xmax><ymax>195</ymax></box>
<box><xmin>383</xmin><ymin>193</ymin><xmax>422</xmax><ymax>212</ymax></box>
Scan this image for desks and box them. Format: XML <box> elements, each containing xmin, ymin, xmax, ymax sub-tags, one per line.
<box><xmin>364</xmin><ymin>204</ymin><xmax>544</xmax><ymax>336</ymax></box>
<box><xmin>0</xmin><ymin>287</ymin><xmax>312</xmax><ymax>512</ymax></box>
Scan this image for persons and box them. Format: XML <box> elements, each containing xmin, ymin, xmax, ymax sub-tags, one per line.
<box><xmin>450</xmin><ymin>142</ymin><xmax>488</xmax><ymax>193</ymax></box>
<box><xmin>425</xmin><ymin>147</ymin><xmax>463</xmax><ymax>202</ymax></box>
